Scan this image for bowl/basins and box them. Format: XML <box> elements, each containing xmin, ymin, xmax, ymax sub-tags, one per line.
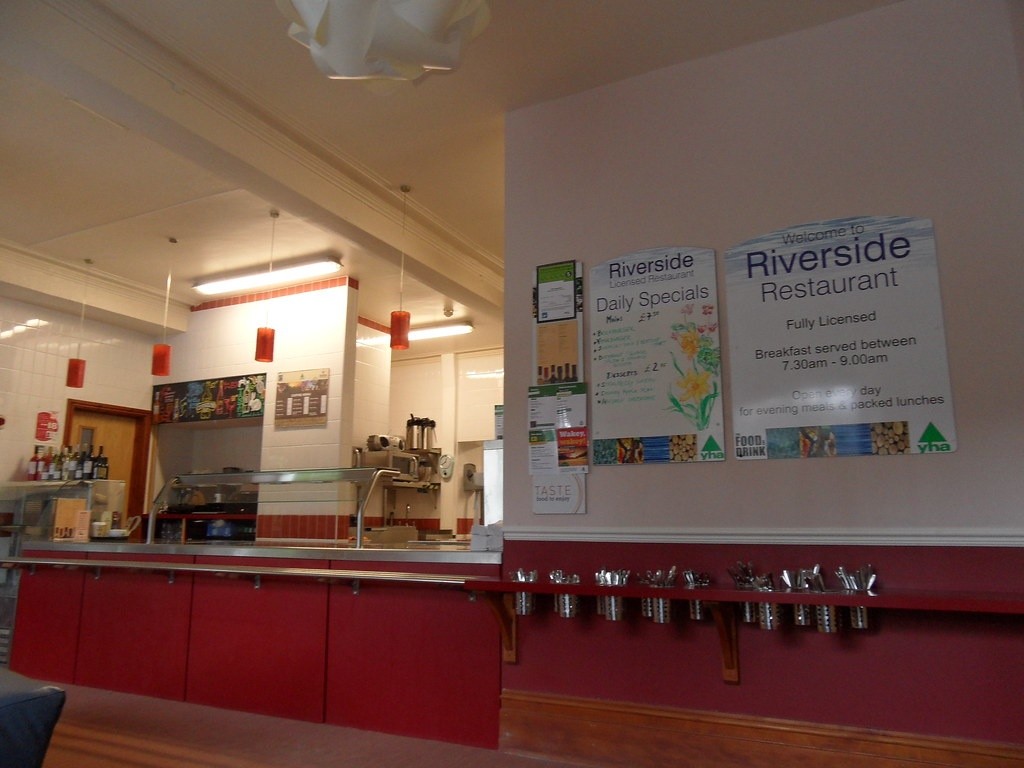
<box><xmin>109</xmin><ymin>529</ymin><xmax>128</xmax><ymax>537</ymax></box>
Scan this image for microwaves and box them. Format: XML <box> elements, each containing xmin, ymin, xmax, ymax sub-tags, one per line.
<box><xmin>361</xmin><ymin>451</ymin><xmax>419</xmax><ymax>482</ymax></box>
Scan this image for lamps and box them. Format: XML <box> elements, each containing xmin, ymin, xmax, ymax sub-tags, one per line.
<box><xmin>151</xmin><ymin>238</ymin><xmax>178</xmax><ymax>376</ymax></box>
<box><xmin>408</xmin><ymin>321</ymin><xmax>476</xmax><ymax>340</ymax></box>
<box><xmin>66</xmin><ymin>259</ymin><xmax>93</xmax><ymax>388</ymax></box>
<box><xmin>390</xmin><ymin>184</ymin><xmax>411</xmax><ymax>350</ymax></box>
<box><xmin>255</xmin><ymin>213</ymin><xmax>279</xmax><ymax>362</ymax></box>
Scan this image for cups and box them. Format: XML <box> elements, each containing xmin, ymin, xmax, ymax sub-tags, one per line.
<box><xmin>92</xmin><ymin>522</ymin><xmax>106</xmax><ymax>538</ymax></box>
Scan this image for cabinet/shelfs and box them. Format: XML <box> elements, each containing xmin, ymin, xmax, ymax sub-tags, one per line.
<box><xmin>10</xmin><ymin>548</ymin><xmax>503</xmax><ymax>751</ymax></box>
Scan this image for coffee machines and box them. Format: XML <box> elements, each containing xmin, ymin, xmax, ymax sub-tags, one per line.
<box><xmin>405</xmin><ymin>413</ymin><xmax>441</xmax><ymax>485</ymax></box>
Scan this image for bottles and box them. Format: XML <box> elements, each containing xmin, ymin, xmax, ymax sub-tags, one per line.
<box><xmin>28</xmin><ymin>444</ymin><xmax>108</xmax><ymax>482</ymax></box>
